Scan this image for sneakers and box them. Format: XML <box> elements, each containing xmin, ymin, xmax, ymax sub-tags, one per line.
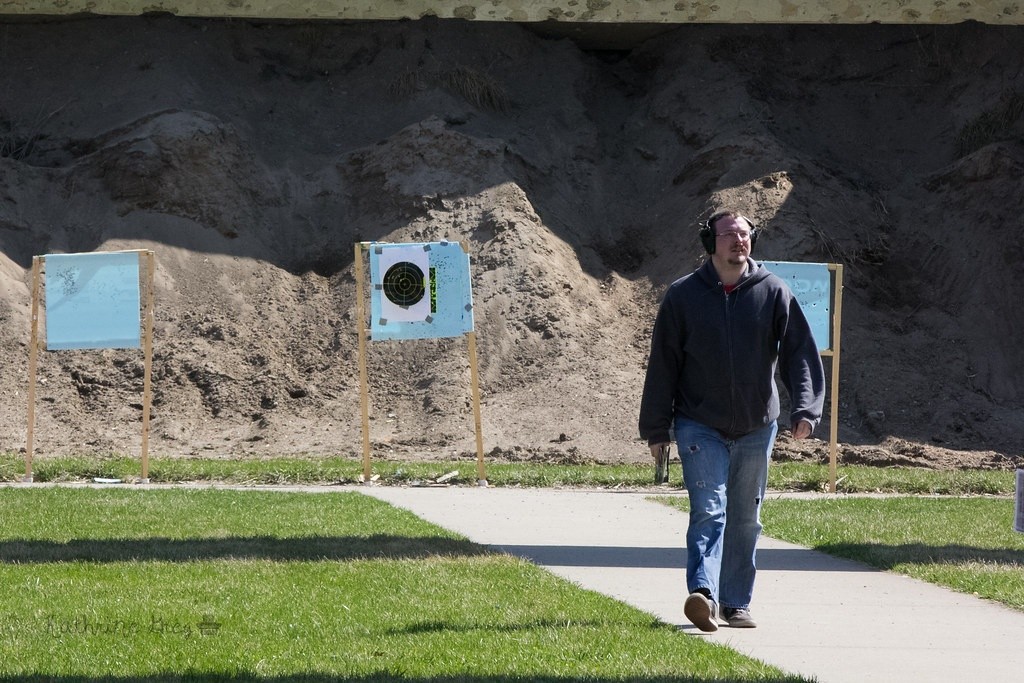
<box><xmin>719</xmin><ymin>604</ymin><xmax>757</xmax><ymax>628</ymax></box>
<box><xmin>684</xmin><ymin>593</ymin><xmax>718</xmax><ymax>632</ymax></box>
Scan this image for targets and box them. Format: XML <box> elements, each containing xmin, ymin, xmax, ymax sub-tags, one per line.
<box><xmin>382</xmin><ymin>261</ymin><xmax>427</xmax><ymax>307</ymax></box>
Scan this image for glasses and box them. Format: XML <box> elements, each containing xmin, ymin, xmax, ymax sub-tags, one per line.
<box><xmin>716</xmin><ymin>231</ymin><xmax>750</xmax><ymax>241</ymax></box>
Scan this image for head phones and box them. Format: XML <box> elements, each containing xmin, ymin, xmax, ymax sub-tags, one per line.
<box><xmin>700</xmin><ymin>212</ymin><xmax>757</xmax><ymax>255</ymax></box>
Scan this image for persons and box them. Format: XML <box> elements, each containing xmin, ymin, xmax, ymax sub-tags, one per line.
<box><xmin>638</xmin><ymin>209</ymin><xmax>826</xmax><ymax>632</ymax></box>
